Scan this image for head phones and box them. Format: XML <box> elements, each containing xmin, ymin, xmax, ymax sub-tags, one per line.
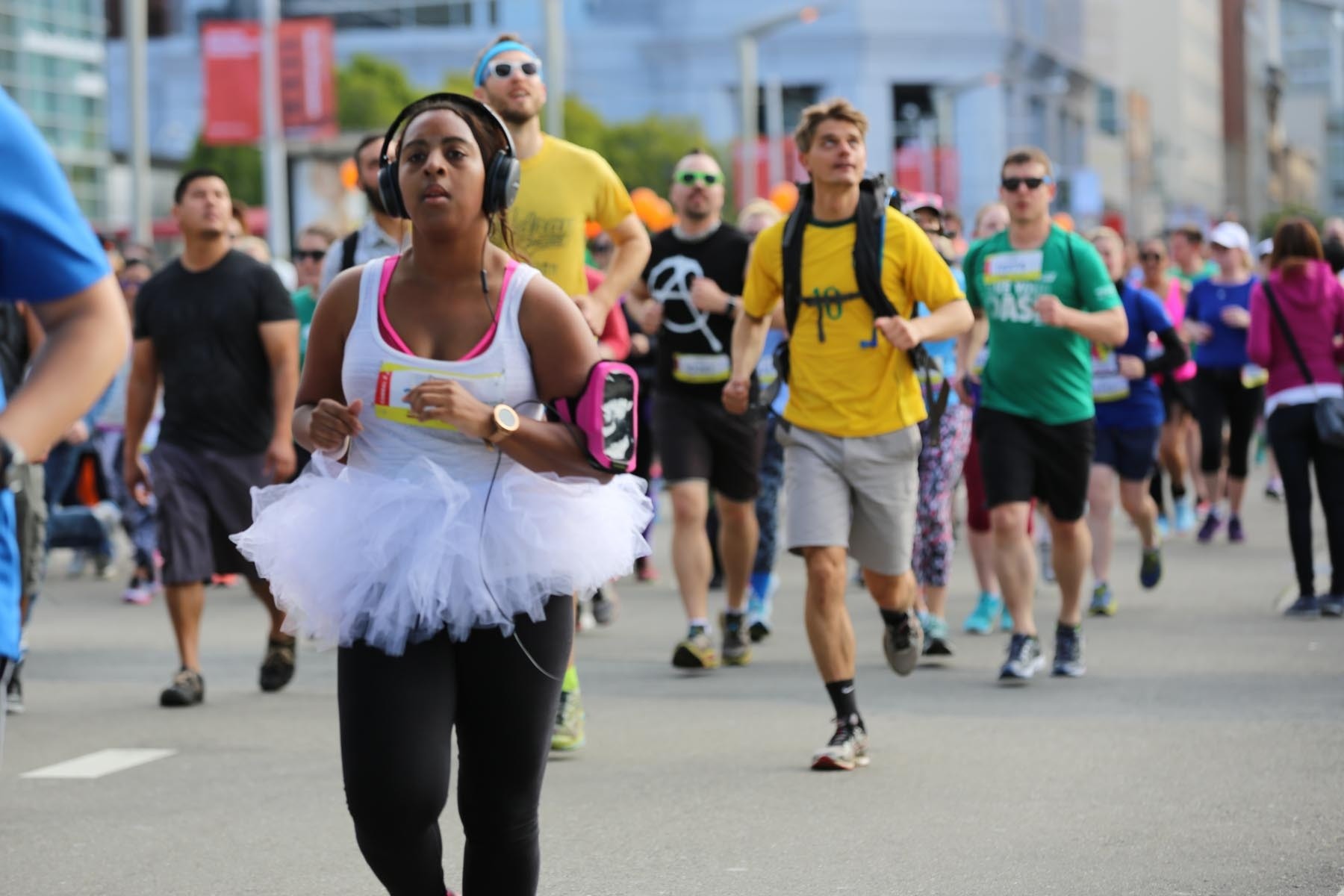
<box><xmin>376</xmin><ymin>92</ymin><xmax>521</xmax><ymax>220</ymax></box>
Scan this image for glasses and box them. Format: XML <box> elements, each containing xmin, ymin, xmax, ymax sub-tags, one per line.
<box><xmin>671</xmin><ymin>172</ymin><xmax>724</xmax><ymax>187</ymax></box>
<box><xmin>1004</xmin><ymin>176</ymin><xmax>1052</xmax><ymax>192</ymax></box>
<box><xmin>484</xmin><ymin>59</ymin><xmax>541</xmax><ymax>80</ymax></box>
<box><xmin>1138</xmin><ymin>251</ymin><xmax>1164</xmax><ymax>263</ymax></box>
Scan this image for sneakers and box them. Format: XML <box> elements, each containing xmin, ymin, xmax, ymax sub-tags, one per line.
<box><xmin>1091</xmin><ymin>584</ymin><xmax>1117</xmax><ymax>617</ymax></box>
<box><xmin>721</xmin><ymin>612</ymin><xmax>751</xmax><ymax>665</ymax></box>
<box><xmin>813</xmin><ymin>725</ymin><xmax>872</xmax><ymax>771</ymax></box>
<box><xmin>552</xmin><ymin>684</ymin><xmax>584</xmax><ymax>751</ymax></box>
<box><xmin>1228</xmin><ymin>517</ymin><xmax>1243</xmax><ymax>543</ymax></box>
<box><xmin>162</xmin><ymin>665</ymin><xmax>205</xmax><ymax>707</ymax></box>
<box><xmin>926</xmin><ymin>619</ymin><xmax>954</xmax><ymax>657</ymax></box>
<box><xmin>1054</xmin><ymin>623</ymin><xmax>1089</xmax><ymax>677</ymax></box>
<box><xmin>1001</xmin><ymin>634</ymin><xmax>1044</xmax><ymax>679</ymax></box>
<box><xmin>122</xmin><ymin>575</ymin><xmax>160</xmax><ymax>607</ymax></box>
<box><xmin>590</xmin><ymin>579</ymin><xmax>620</xmax><ymax>625</ymax></box>
<box><xmin>1266</xmin><ymin>478</ymin><xmax>1283</xmax><ymax>502</ymax></box>
<box><xmin>262</xmin><ymin>634</ymin><xmax>295</xmax><ymax>689</ymax></box>
<box><xmin>1174</xmin><ymin>500</ymin><xmax>1193</xmax><ymax>534</ymax></box>
<box><xmin>1155</xmin><ymin>514</ymin><xmax>1170</xmax><ymax>539</ymax></box>
<box><xmin>1318</xmin><ymin>594</ymin><xmax>1344</xmax><ymax>615</ymax></box>
<box><xmin>1140</xmin><ymin>542</ymin><xmax>1164</xmax><ymax>589</ymax></box>
<box><xmin>1198</xmin><ymin>515</ymin><xmax>1221</xmax><ymax>543</ymax></box>
<box><xmin>962</xmin><ymin>592</ymin><xmax>999</xmax><ymax>633</ymax></box>
<box><xmin>881</xmin><ymin>610</ymin><xmax>925</xmax><ymax>676</ymax></box>
<box><xmin>748</xmin><ymin>574</ymin><xmax>778</xmax><ymax>642</ymax></box>
<box><xmin>674</xmin><ymin>625</ymin><xmax>720</xmax><ymax>670</ymax></box>
<box><xmin>1286</xmin><ymin>598</ymin><xmax>1322</xmax><ymax>619</ymax></box>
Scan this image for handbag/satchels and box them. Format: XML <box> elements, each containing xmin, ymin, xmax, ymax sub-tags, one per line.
<box><xmin>1310</xmin><ymin>398</ymin><xmax>1343</xmax><ymax>446</ymax></box>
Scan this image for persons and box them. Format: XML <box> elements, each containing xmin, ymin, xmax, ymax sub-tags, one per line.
<box><xmin>0</xmin><ymin>31</ymin><xmax>1344</xmax><ymax>774</ymax></box>
<box><xmin>227</xmin><ymin>94</ymin><xmax>658</xmax><ymax>896</ymax></box>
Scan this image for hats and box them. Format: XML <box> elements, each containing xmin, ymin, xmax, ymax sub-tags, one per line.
<box><xmin>1255</xmin><ymin>239</ymin><xmax>1274</xmax><ymax>257</ymax></box>
<box><xmin>1203</xmin><ymin>222</ymin><xmax>1250</xmax><ymax>251</ymax></box>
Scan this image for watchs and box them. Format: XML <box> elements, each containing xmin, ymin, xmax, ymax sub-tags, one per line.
<box><xmin>483</xmin><ymin>404</ymin><xmax>520</xmax><ymax>449</ymax></box>
